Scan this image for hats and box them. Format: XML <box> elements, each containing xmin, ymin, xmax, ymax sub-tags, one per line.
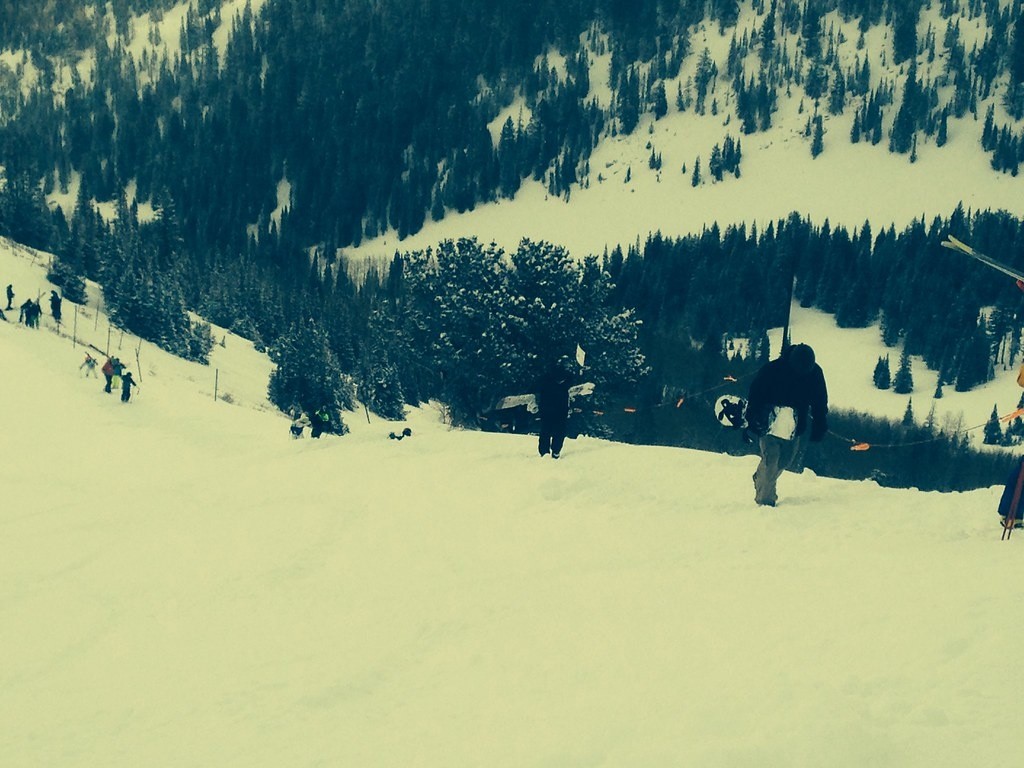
<box><xmin>791</xmin><ymin>342</ymin><xmax>815</xmax><ymax>377</ymax></box>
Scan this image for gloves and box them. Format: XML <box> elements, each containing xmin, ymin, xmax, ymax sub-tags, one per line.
<box><xmin>749</xmin><ymin>420</ymin><xmax>770</xmax><ymax>436</ymax></box>
<box><xmin>810</xmin><ymin>429</ymin><xmax>827</xmax><ymax>442</ymax></box>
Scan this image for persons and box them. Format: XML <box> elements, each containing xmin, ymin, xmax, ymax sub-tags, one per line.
<box><xmin>312</xmin><ymin>406</ymin><xmax>329</xmax><ymax>439</ymax></box>
<box><xmin>289</xmin><ymin>416</ymin><xmax>312</xmax><ymax>439</ymax></box>
<box><xmin>49</xmin><ymin>290</ymin><xmax>62</xmax><ymax>323</ymax></box>
<box><xmin>535</xmin><ymin>364</ymin><xmax>572</xmax><ymax>459</ymax></box>
<box><xmin>101</xmin><ymin>357</ymin><xmax>137</xmax><ymax>404</ymax></box>
<box><xmin>19</xmin><ymin>299</ymin><xmax>42</xmax><ymax>329</ymax></box>
<box><xmin>743</xmin><ymin>344</ymin><xmax>829</xmax><ymax>507</ymax></box>
<box><xmin>80</xmin><ymin>356</ymin><xmax>98</xmax><ymax>379</ymax></box>
<box><xmin>5</xmin><ymin>284</ymin><xmax>15</xmax><ymax>310</ymax></box>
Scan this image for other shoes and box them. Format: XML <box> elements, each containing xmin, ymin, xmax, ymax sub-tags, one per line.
<box><xmin>755</xmin><ymin>498</ymin><xmax>775</xmax><ymax>507</ymax></box>
<box><xmin>752</xmin><ymin>473</ymin><xmax>758</xmax><ymax>489</ymax></box>
<box><xmin>1000</xmin><ymin>517</ymin><xmax>1024</xmax><ymax>530</ymax></box>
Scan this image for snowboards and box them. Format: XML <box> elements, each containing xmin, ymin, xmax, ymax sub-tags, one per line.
<box><xmin>496</xmin><ymin>380</ymin><xmax>596</xmax><ymax>412</ymax></box>
<box><xmin>714</xmin><ymin>394</ymin><xmax>801</xmax><ymax>442</ymax></box>
